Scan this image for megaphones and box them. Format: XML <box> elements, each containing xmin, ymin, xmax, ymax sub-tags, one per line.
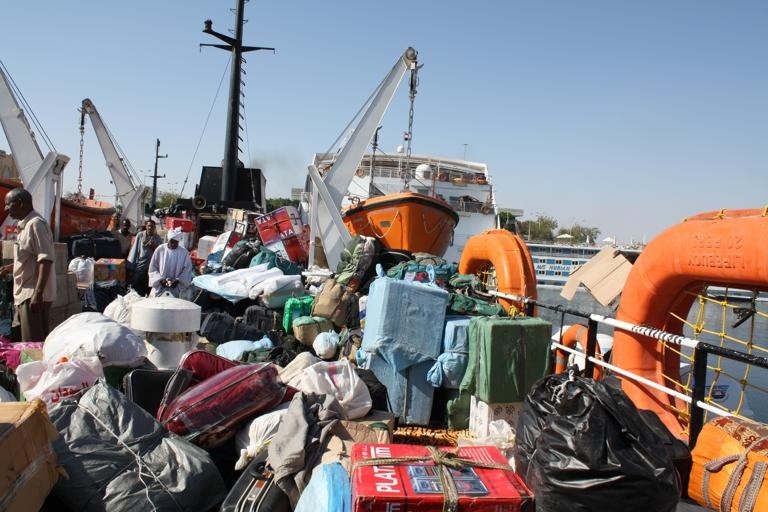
<box><xmin>192</xmin><ymin>195</ymin><xmax>207</xmax><ymax>210</ymax></box>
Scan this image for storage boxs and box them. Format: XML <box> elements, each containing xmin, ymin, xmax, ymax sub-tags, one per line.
<box><xmin>197</xmin><ymin>235</ymin><xmax>217</xmax><ymax>260</ymax></box>
<box><xmin>167</xmin><ymin>215</ymin><xmax>194</xmax><ymax>250</ymax></box>
<box><xmin>350</xmin><ymin>442</ymin><xmax>536</xmax><ymax>512</ymax></box>
<box><xmin>0</xmin><ymin>240</ymin><xmax>82</xmax><ymax>335</ymax></box>
<box><xmin>254</xmin><ymin>205</ymin><xmax>310</xmax><ymax>263</ymax></box>
<box><xmin>358</xmin><ymin>276</ymin><xmax>553</xmax><ymax>439</ymax></box>
<box><xmin>93</xmin><ymin>257</ymin><xmax>127</xmax><ymax>288</ymax></box>
<box><xmin>0</xmin><ymin>397</ymin><xmax>59</xmax><ymax>512</ymax></box>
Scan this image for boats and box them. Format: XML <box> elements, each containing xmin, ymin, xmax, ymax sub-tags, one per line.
<box><xmin>0</xmin><ymin>177</ymin><xmax>117</xmax><ymax>237</ymax></box>
<box><xmin>335</xmin><ymin>189</ymin><xmax>460</xmax><ymax>264</ymax></box>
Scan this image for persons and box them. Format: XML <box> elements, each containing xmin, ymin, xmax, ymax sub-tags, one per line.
<box><xmin>117</xmin><ymin>218</ymin><xmax>192</xmax><ymax>298</ymax></box>
<box><xmin>0</xmin><ymin>188</ymin><xmax>58</xmax><ymax>343</ymax></box>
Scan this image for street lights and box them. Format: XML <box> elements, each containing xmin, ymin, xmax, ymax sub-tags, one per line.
<box><xmin>138</xmin><ymin>168</ymin><xmax>152</xmax><ymax>184</ymax></box>
<box><xmin>167</xmin><ymin>180</ymin><xmax>179</xmax><ymax>201</ymax></box>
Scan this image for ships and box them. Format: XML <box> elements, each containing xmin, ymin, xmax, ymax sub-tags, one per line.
<box><xmin>302</xmin><ymin>138</ymin><xmax>502</xmax><ymax>276</ymax></box>
<box><xmin>520</xmin><ymin>240</ymin><xmax>768</xmax><ymax>304</ymax></box>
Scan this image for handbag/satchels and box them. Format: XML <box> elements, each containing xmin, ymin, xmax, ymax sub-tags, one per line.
<box><xmin>638</xmin><ymin>407</ymin><xmax>691</xmax><ymax>475</ymax></box>
<box><xmin>220</xmin><ymin>443</ymin><xmax>288</xmax><ymax>512</ymax></box>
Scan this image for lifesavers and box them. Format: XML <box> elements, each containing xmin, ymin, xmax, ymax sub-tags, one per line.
<box><xmin>555</xmin><ymin>327</ymin><xmax>603</xmax><ymax>380</ymax></box>
<box><xmin>356</xmin><ymin>168</ymin><xmax>365</xmax><ymax>177</ymax></box>
<box><xmin>351</xmin><ymin>197</ymin><xmax>360</xmax><ymax>204</ymax></box>
